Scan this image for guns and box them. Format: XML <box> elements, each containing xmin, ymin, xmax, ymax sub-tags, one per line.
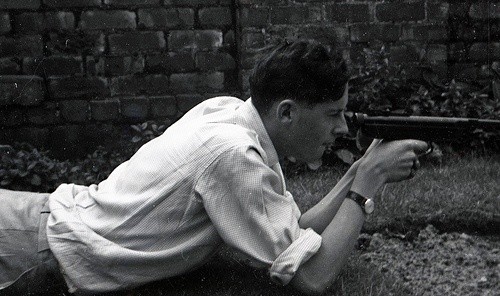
<box><xmin>355</xmin><ymin>111</ymin><xmax>500</xmax><ymax>170</ymax></box>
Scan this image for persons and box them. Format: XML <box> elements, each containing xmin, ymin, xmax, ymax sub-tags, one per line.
<box><xmin>0</xmin><ymin>41</ymin><xmax>429</xmax><ymax>296</ymax></box>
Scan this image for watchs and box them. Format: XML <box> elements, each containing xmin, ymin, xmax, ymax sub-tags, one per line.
<box><xmin>345</xmin><ymin>189</ymin><xmax>375</xmax><ymax>214</ymax></box>
